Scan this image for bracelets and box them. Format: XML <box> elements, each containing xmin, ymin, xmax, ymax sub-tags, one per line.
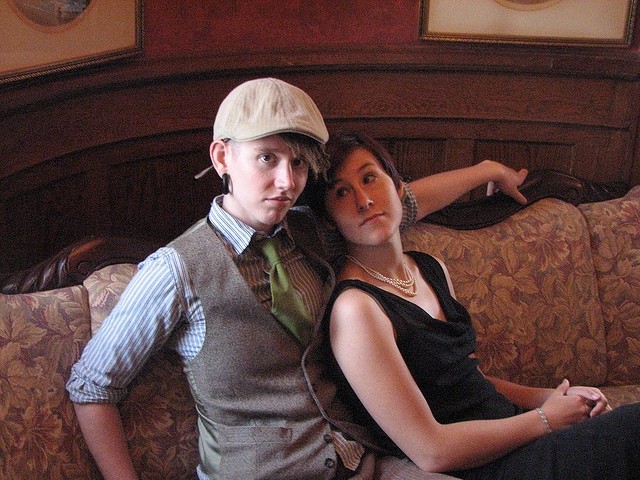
<box><xmin>535</xmin><ymin>407</ymin><xmax>552</xmax><ymax>435</ymax></box>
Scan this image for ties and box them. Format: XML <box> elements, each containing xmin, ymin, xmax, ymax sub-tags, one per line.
<box><xmin>259</xmin><ymin>238</ymin><xmax>315</xmax><ymax>347</ymax></box>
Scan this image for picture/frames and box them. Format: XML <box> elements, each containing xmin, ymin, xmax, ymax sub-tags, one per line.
<box><xmin>1</xmin><ymin>1</ymin><xmax>145</xmax><ymax>86</ymax></box>
<box><xmin>414</xmin><ymin>0</ymin><xmax>639</xmax><ymax>49</ymax></box>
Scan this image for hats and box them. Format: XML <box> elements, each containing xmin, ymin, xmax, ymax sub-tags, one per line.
<box><xmin>193</xmin><ymin>78</ymin><xmax>330</xmax><ymax>179</ymax></box>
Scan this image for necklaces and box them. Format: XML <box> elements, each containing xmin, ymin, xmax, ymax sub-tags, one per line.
<box><xmin>345</xmin><ymin>253</ymin><xmax>419</xmax><ymax>297</ymax></box>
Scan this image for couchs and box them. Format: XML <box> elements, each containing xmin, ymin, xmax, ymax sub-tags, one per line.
<box><xmin>2</xmin><ymin>164</ymin><xmax>640</xmax><ymax>479</ymax></box>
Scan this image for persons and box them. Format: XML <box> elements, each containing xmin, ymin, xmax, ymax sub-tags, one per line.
<box><xmin>315</xmin><ymin>128</ymin><xmax>640</xmax><ymax>480</ymax></box>
<box><xmin>66</xmin><ymin>78</ymin><xmax>528</xmax><ymax>480</ymax></box>
